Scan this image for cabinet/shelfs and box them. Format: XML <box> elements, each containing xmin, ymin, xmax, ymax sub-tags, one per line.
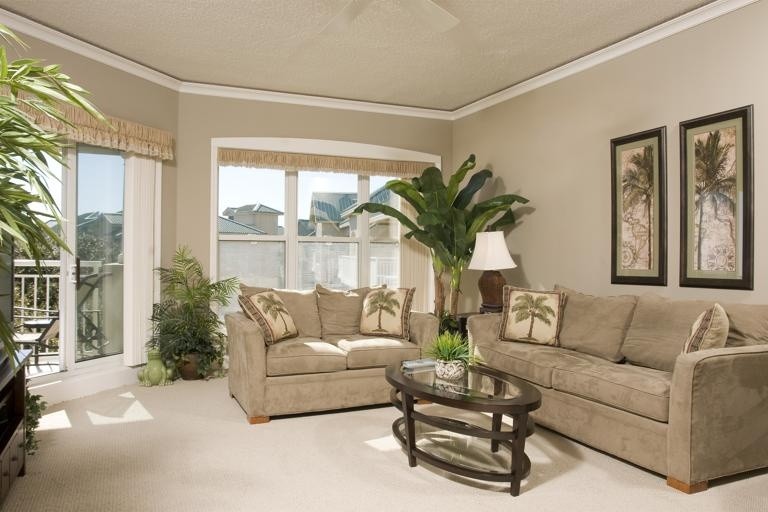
<box><xmin>0</xmin><ymin>349</ymin><xmax>34</xmax><ymax>508</ymax></box>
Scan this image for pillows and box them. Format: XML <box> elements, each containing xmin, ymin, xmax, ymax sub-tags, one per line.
<box><xmin>234</xmin><ymin>290</ymin><xmax>299</xmax><ymax>345</ymax></box>
<box><xmin>239</xmin><ymin>283</ymin><xmax>322</xmax><ymax>339</ymax></box>
<box><xmin>316</xmin><ymin>283</ymin><xmax>388</xmax><ymax>338</ymax></box>
<box><xmin>360</xmin><ymin>286</ymin><xmax>416</xmax><ymax>341</ymax></box>
<box><xmin>621</xmin><ymin>293</ymin><xmax>767</xmax><ymax>372</ymax></box>
<box><xmin>498</xmin><ymin>283</ymin><xmax>567</xmax><ymax>347</ymax></box>
<box><xmin>546</xmin><ymin>284</ymin><xmax>639</xmax><ymax>363</ymax></box>
<box><xmin>679</xmin><ymin>302</ymin><xmax>730</xmax><ymax>353</ymax></box>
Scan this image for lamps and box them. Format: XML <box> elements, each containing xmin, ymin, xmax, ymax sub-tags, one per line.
<box><xmin>465</xmin><ymin>230</ymin><xmax>517</xmax><ymax>314</ymax></box>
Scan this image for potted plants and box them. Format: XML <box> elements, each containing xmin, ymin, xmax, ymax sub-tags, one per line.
<box><xmin>143</xmin><ymin>244</ymin><xmax>243</xmax><ymax>381</ymax></box>
<box><xmin>421</xmin><ymin>329</ymin><xmax>486</xmax><ymax>380</ymax></box>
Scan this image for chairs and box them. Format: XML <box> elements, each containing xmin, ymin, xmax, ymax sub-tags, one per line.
<box><xmin>10</xmin><ymin>270</ymin><xmax>115</xmax><ymax>373</ymax></box>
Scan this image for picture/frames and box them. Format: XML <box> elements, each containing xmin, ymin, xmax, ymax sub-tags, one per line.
<box><xmin>677</xmin><ymin>104</ymin><xmax>756</xmax><ymax>291</ymax></box>
<box><xmin>609</xmin><ymin>125</ymin><xmax>669</xmax><ymax>287</ymax></box>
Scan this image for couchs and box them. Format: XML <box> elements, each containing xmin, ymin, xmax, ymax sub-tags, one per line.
<box><xmin>466</xmin><ymin>285</ymin><xmax>767</xmax><ymax>494</ymax></box>
<box><xmin>224</xmin><ymin>283</ymin><xmax>440</xmax><ymax>425</ymax></box>
